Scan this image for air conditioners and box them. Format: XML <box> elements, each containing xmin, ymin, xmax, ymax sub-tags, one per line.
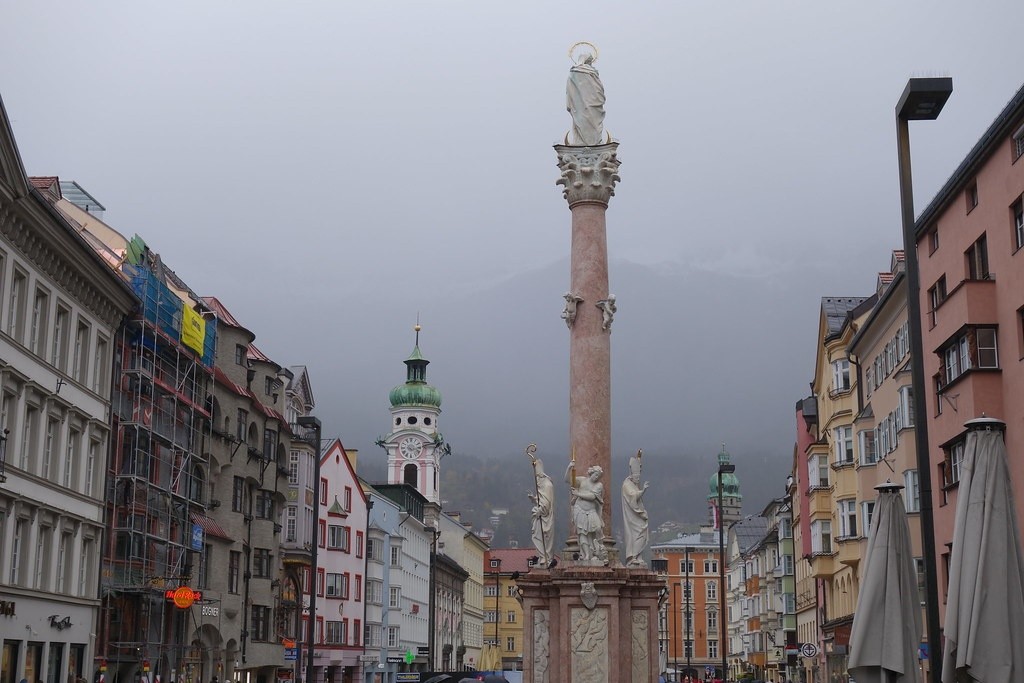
<box><xmin>211</xmin><ymin>500</ymin><xmax>221</xmax><ymax>508</ymax></box>
<box><xmin>275</xmin><ymin>526</ymin><xmax>282</xmax><ymax>533</ymax></box>
<box><xmin>244</xmin><ymin>513</ymin><xmax>253</xmax><ymax>521</ymax></box>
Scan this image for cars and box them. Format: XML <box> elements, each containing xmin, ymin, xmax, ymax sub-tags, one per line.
<box><xmin>420</xmin><ymin>670</ymin><xmax>512</xmax><ymax>683</ymax></box>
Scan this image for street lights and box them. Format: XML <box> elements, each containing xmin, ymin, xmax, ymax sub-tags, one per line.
<box><xmin>294</xmin><ymin>414</ymin><xmax>321</xmax><ymax>683</ymax></box>
<box><xmin>717</xmin><ymin>464</ymin><xmax>737</xmax><ymax>683</ymax></box>
<box><xmin>491</xmin><ymin>572</ymin><xmax>499</xmax><ymax>649</ymax></box>
<box><xmin>423</xmin><ymin>526</ymin><xmax>435</xmax><ymax>673</ymax></box>
<box><xmin>673</xmin><ymin>582</ymin><xmax>680</xmax><ymax>682</ymax></box>
<box><xmin>894</xmin><ymin>76</ymin><xmax>954</xmax><ymax>683</ymax></box>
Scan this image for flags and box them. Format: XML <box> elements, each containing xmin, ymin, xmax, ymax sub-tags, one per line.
<box><xmin>709</xmin><ymin>501</ymin><xmax>719</xmax><ymax>531</ymax></box>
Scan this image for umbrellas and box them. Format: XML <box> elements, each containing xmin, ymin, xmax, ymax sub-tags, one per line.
<box><xmin>476</xmin><ymin>640</ymin><xmax>502</xmax><ymax>671</ymax></box>
<box><xmin>659</xmin><ymin>650</ymin><xmax>666</xmax><ymax>674</ymax></box>
<box><xmin>941</xmin><ymin>412</ymin><xmax>1024</xmax><ymax>683</ymax></box>
<box><xmin>847</xmin><ymin>479</ymin><xmax>922</xmax><ymax>683</ymax></box>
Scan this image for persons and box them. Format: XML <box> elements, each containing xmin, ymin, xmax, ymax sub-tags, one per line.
<box><xmin>210</xmin><ymin>676</ymin><xmax>218</xmax><ymax>683</ymax></box>
<box><xmin>563</xmin><ymin>461</ymin><xmax>604</xmax><ymax>560</ymax></box>
<box><xmin>562</xmin><ymin>292</ymin><xmax>584</xmax><ymax>330</ymax></box>
<box><xmin>621</xmin><ymin>457</ymin><xmax>649</xmax><ymax>564</ymax></box>
<box><xmin>76</xmin><ymin>674</ymin><xmax>88</xmax><ymax>683</ymax></box>
<box><xmin>596</xmin><ymin>294</ymin><xmax>617</xmax><ymax>329</ymax></box>
<box><xmin>526</xmin><ymin>459</ymin><xmax>555</xmax><ymax>564</ymax></box>
<box><xmin>566</xmin><ymin>40</ymin><xmax>607</xmax><ymax>146</ymax></box>
<box><xmin>684</xmin><ymin>674</ymin><xmax>693</xmax><ymax>683</ymax></box>
<box><xmin>739</xmin><ymin>675</ymin><xmax>774</xmax><ymax>683</ymax></box>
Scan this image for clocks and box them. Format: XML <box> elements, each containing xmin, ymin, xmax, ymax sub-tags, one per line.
<box><xmin>398</xmin><ymin>436</ymin><xmax>423</xmax><ymax>460</ymax></box>
